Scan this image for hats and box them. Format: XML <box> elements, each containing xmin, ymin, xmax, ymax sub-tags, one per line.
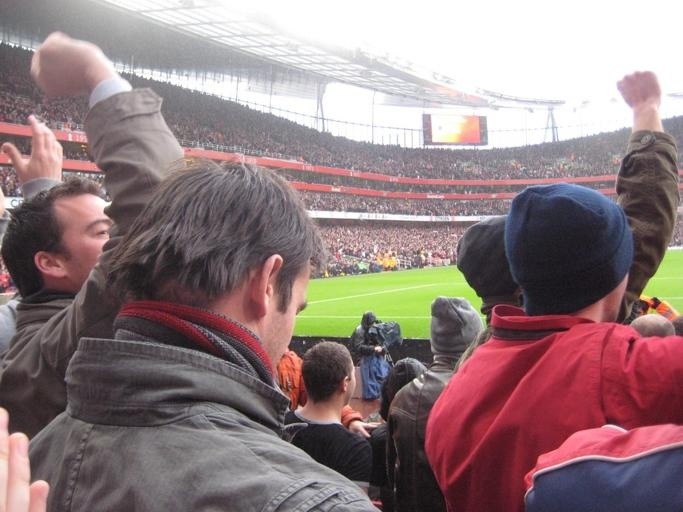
<box><xmin>502</xmin><ymin>179</ymin><xmax>635</xmax><ymax>320</ymax></box>
<box><xmin>455</xmin><ymin>215</ymin><xmax>517</xmax><ymax>310</ymax></box>
<box><xmin>427</xmin><ymin>296</ymin><xmax>483</xmax><ymax>358</ymax></box>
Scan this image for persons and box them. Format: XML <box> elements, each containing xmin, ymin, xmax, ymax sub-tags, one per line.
<box><xmin>0</xmin><ymin>30</ymin><xmax>682</xmax><ymax>510</ymax></box>
